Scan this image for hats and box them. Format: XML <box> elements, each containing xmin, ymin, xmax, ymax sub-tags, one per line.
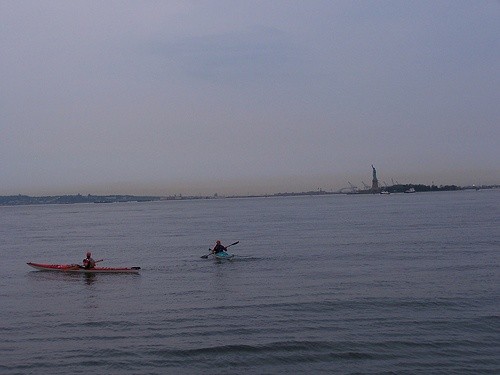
<box><xmin>216</xmin><ymin>240</ymin><xmax>220</xmax><ymax>243</ymax></box>
<box><xmin>87</xmin><ymin>252</ymin><xmax>91</xmax><ymax>256</ymax></box>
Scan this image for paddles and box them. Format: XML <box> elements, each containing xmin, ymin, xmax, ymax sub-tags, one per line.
<box><xmin>200</xmin><ymin>241</ymin><xmax>239</xmax><ymax>259</ymax></box>
<box><xmin>64</xmin><ymin>259</ymin><xmax>104</xmax><ymax>270</ymax></box>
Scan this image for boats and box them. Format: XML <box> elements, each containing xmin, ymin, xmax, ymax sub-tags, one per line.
<box><xmin>403</xmin><ymin>188</ymin><xmax>415</xmax><ymax>193</ymax></box>
<box><xmin>380</xmin><ymin>191</ymin><xmax>390</xmax><ymax>194</ymax></box>
<box><xmin>27</xmin><ymin>262</ymin><xmax>142</xmax><ymax>275</ymax></box>
<box><xmin>208</xmin><ymin>248</ymin><xmax>235</xmax><ymax>259</ymax></box>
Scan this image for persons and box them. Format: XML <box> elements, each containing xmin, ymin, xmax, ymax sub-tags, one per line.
<box><xmin>83</xmin><ymin>252</ymin><xmax>97</xmax><ymax>268</ymax></box>
<box><xmin>212</xmin><ymin>240</ymin><xmax>227</xmax><ymax>254</ymax></box>
